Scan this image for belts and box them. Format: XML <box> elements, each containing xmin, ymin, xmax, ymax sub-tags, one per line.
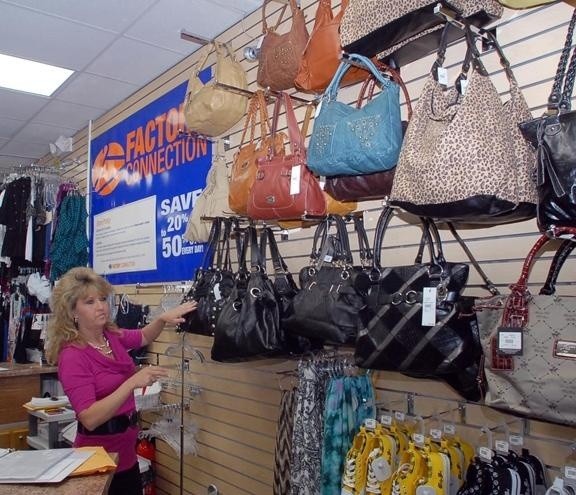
<box><xmin>78</xmin><ymin>411</ymin><xmax>138</xmax><ymax>435</ymax></box>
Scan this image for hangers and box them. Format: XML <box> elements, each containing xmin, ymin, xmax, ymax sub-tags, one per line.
<box><xmin>164</xmin><ymin>326</ymin><xmax>207</xmax><ymax>366</ymax></box>
<box><xmin>136</xmin><ymin>404</ymin><xmax>200</xmax><ymax>461</ymax></box>
<box><xmin>142</xmin><ymin>281</ymin><xmax>187</xmax><ymax>332</ymax></box>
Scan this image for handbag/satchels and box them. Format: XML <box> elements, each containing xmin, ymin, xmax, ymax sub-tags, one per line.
<box><xmin>517</xmin><ymin>9</ymin><xmax>576</xmax><ymax>229</ymax></box>
<box><xmin>181</xmin><ymin>0</ymin><xmax>412</xmax><ymax>223</ymax></box>
<box><xmin>413</xmin><ymin>0</ymin><xmax>505</xmax><ymax>64</ymax></box>
<box><xmin>387</xmin><ymin>20</ymin><xmax>540</xmax><ymax>221</ymax></box>
<box><xmin>473</xmin><ymin>268</ymin><xmax>575</xmax><ymax>424</ymax></box>
<box><xmin>176</xmin><ymin>266</ymin><xmax>481</xmax><ymax>402</ymax></box>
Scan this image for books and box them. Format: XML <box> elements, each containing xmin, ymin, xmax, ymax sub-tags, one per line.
<box><xmin>0</xmin><ymin>447</ymin><xmax>74</xmax><ymax>481</ymax></box>
<box><xmin>25</xmin><ymin>378</ymin><xmax>69</xmax><ymax>412</ymax></box>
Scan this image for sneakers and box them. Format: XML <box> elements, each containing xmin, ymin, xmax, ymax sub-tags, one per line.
<box><xmin>340</xmin><ymin>426</ymin><xmax>546</xmax><ymax>493</ymax></box>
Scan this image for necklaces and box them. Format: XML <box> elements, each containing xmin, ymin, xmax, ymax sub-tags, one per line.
<box><xmin>104</xmin><ymin>350</ymin><xmax>112</xmax><ymax>355</ymax></box>
<box><xmin>86</xmin><ymin>333</ymin><xmax>109</xmax><ymax>351</ymax></box>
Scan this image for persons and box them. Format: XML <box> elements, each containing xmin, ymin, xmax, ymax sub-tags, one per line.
<box><xmin>42</xmin><ymin>265</ymin><xmax>198</xmax><ymax>495</ymax></box>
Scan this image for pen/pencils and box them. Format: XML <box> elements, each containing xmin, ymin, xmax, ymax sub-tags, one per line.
<box><xmin>142</xmin><ymin>364</ymin><xmax>152</xmax><ymax>396</ymax></box>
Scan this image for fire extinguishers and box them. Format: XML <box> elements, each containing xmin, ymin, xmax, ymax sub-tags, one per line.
<box><xmin>136</xmin><ymin>437</ymin><xmax>156</xmax><ymax>495</ymax></box>
